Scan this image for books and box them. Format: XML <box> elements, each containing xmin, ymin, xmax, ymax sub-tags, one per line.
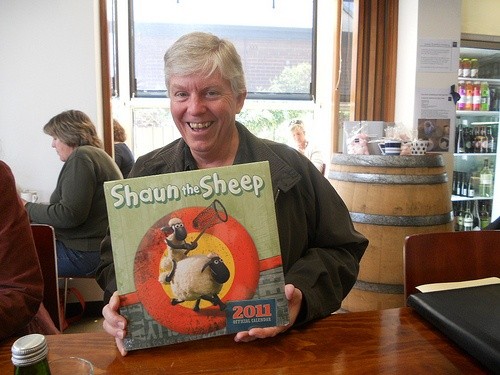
<box><xmin>103</xmin><ymin>161</ymin><xmax>289</xmax><ymax>350</ymax></box>
<box><xmin>409</xmin><ymin>277</ymin><xmax>500</xmax><ymax>375</ymax></box>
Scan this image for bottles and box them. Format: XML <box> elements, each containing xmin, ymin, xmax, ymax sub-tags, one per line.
<box><xmin>458</xmin><ymin>58</ymin><xmax>478</xmax><ymax>78</ymax></box>
<box><xmin>451</xmin><ymin>201</ymin><xmax>490</xmax><ymax>232</ymax></box>
<box><xmin>11</xmin><ymin>333</ymin><xmax>51</xmax><ymax>375</ymax></box>
<box><xmin>479</xmin><ymin>158</ymin><xmax>492</xmax><ymax>198</ymax></box>
<box><xmin>457</xmin><ymin>80</ymin><xmax>490</xmax><ymax>111</ymax></box>
<box><xmin>456</xmin><ymin>124</ymin><xmax>494</xmax><ymax>153</ymax></box>
<box><xmin>452</xmin><ymin>170</ymin><xmax>475</xmax><ymax>197</ymax></box>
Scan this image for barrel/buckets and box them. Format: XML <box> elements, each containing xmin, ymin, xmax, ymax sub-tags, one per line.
<box><xmin>329</xmin><ymin>152</ymin><xmax>455</xmax><ymax>293</ymax></box>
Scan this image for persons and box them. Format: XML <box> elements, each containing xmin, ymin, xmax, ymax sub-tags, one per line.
<box><xmin>95</xmin><ymin>32</ymin><xmax>370</xmax><ymax>356</ymax></box>
<box><xmin>22</xmin><ymin>110</ymin><xmax>124</xmax><ymax>330</ymax></box>
<box><xmin>0</xmin><ymin>160</ymin><xmax>44</xmax><ymax>342</ymax></box>
<box><xmin>112</xmin><ymin>120</ymin><xmax>134</xmax><ymax>178</ymax></box>
<box><xmin>285</xmin><ymin>118</ymin><xmax>323</xmax><ymax>173</ymax></box>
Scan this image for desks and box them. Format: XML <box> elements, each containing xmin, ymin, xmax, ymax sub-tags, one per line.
<box><xmin>0</xmin><ymin>304</ymin><xmax>493</xmax><ymax>375</ymax></box>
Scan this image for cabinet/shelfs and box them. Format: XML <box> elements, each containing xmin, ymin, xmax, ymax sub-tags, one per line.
<box><xmin>450</xmin><ymin>76</ymin><xmax>500</xmax><ymax>233</ymax></box>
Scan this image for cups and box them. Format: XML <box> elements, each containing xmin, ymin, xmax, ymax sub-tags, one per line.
<box><xmin>410</xmin><ymin>141</ymin><xmax>427</xmax><ymax>155</ymax></box>
<box><xmin>49</xmin><ymin>357</ymin><xmax>95</xmax><ymax>375</ymax></box>
<box><xmin>378</xmin><ymin>141</ymin><xmax>401</xmax><ymax>155</ymax></box>
<box><xmin>20</xmin><ymin>191</ymin><xmax>38</xmax><ymax>203</ymax></box>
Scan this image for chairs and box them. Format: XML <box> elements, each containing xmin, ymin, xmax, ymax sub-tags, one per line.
<box><xmin>29</xmin><ymin>223</ymin><xmax>99</xmax><ymax>333</ymax></box>
<box><xmin>403</xmin><ymin>230</ymin><xmax>500</xmax><ymax>307</ymax></box>
